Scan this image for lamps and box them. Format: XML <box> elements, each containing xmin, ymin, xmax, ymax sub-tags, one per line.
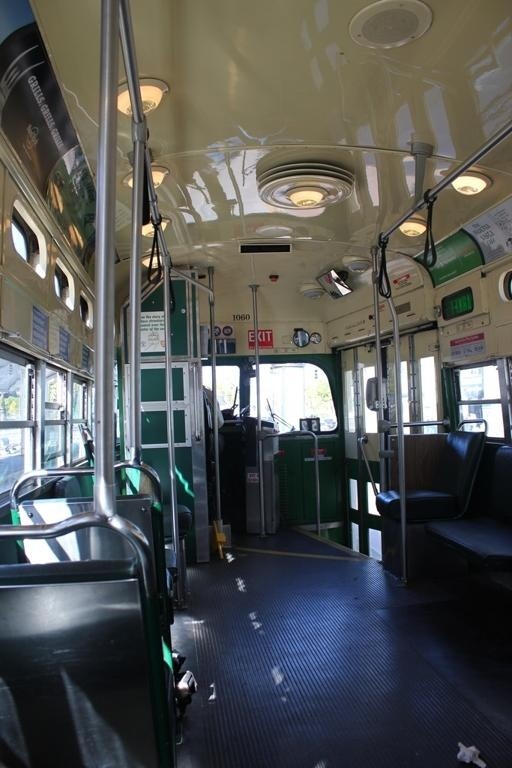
<box><xmin>255</xmin><ymin>158</ymin><xmax>357</xmax><ymax>214</ymax></box>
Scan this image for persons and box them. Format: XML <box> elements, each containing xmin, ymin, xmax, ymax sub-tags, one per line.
<box><xmin>201</xmin><ymin>385</ymin><xmax>226</xmax><ymax>458</ymax></box>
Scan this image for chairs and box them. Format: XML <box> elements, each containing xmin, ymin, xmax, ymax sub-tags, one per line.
<box><xmin>375</xmin><ymin>413</ymin><xmax>512</xmax><ymax>609</ymax></box>
<box><xmin>0</xmin><ymin>436</ymin><xmax>191</xmax><ymax>768</ymax></box>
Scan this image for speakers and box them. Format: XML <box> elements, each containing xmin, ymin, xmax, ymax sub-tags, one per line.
<box><xmin>239</xmin><ymin>244</ymin><xmax>292</xmax><ymax>254</ymax></box>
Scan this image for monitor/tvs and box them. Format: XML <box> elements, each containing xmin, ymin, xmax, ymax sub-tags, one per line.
<box><xmin>317</xmin><ymin>268</ymin><xmax>353</xmax><ymax>300</ymax></box>
